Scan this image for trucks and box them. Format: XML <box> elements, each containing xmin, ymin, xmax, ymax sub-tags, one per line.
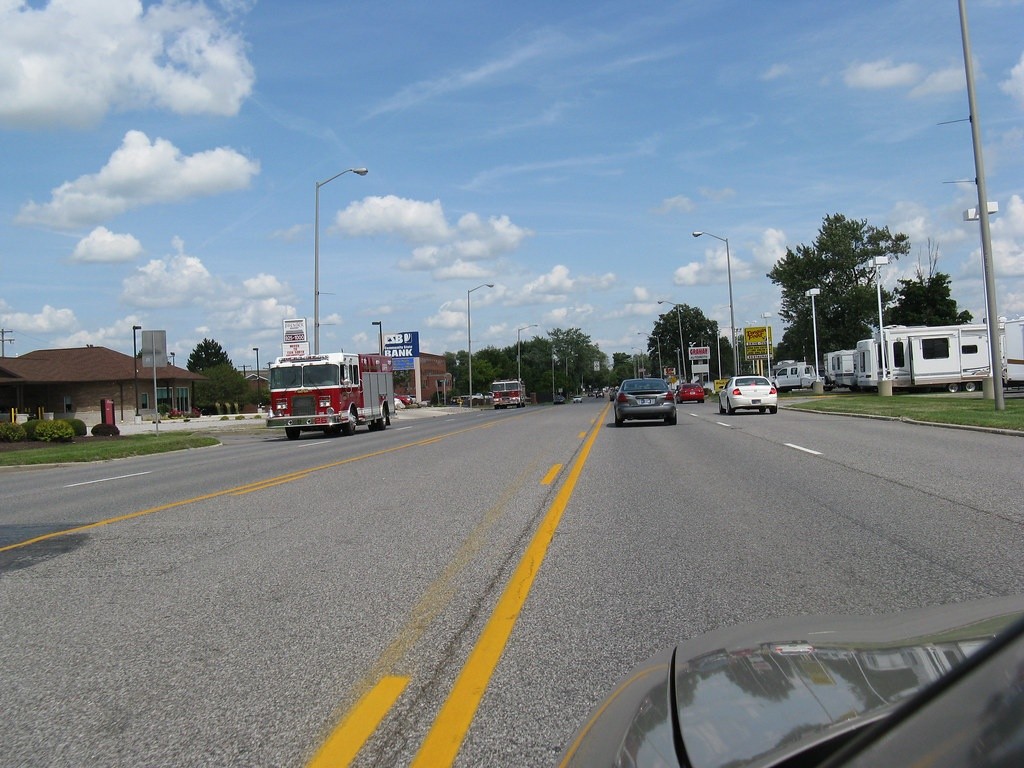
<box><xmin>823</xmin><ymin>316</ymin><xmax>1024</xmax><ymax>393</ymax></box>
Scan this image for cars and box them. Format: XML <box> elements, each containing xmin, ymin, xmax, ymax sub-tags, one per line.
<box><xmin>718</xmin><ymin>376</ymin><xmax>778</xmax><ymax>414</ymax></box>
<box><xmin>610</xmin><ymin>374</ymin><xmax>705</xmax><ymax>425</ymax></box>
<box><xmin>553</xmin><ymin>388</ymin><xmax>604</xmax><ymax>405</ymax></box>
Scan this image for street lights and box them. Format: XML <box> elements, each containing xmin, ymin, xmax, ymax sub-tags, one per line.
<box><xmin>761</xmin><ymin>312</ymin><xmax>772</xmax><ymax>379</ymax></box>
<box><xmin>372</xmin><ymin>321</ymin><xmax>382</xmax><ymax>355</ymax></box>
<box><xmin>638</xmin><ymin>332</ymin><xmax>662</xmax><ymax>378</ymax></box>
<box><xmin>468</xmin><ymin>283</ymin><xmax>579</xmax><ymax>408</ymax></box>
<box><xmin>866</xmin><ymin>256</ymin><xmax>888</xmax><ymax>379</ymax></box>
<box><xmin>133</xmin><ymin>326</ymin><xmax>142</xmax><ymax>416</ymax></box>
<box><xmin>253</xmin><ymin>347</ymin><xmax>262</xmax><ymax>407</ymax></box>
<box><xmin>964</xmin><ymin>202</ymin><xmax>998</xmax><ymax>377</ymax></box>
<box><xmin>693</xmin><ymin>231</ymin><xmax>737</xmax><ymax>376</ymax></box>
<box><xmin>314</xmin><ymin>168</ymin><xmax>369</xmax><ymax>354</ymax></box>
<box><xmin>658</xmin><ymin>300</ymin><xmax>686</xmax><ymax>383</ymax></box>
<box><xmin>631</xmin><ymin>347</ymin><xmax>644</xmax><ymax>378</ymax></box>
<box><xmin>734</xmin><ymin>327</ymin><xmax>741</xmax><ymax>376</ymax></box>
<box><xmin>805</xmin><ymin>288</ymin><xmax>821</xmax><ymax>381</ymax></box>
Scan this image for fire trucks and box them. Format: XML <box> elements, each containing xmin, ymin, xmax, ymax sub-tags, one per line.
<box><xmin>266</xmin><ymin>353</ymin><xmax>397</xmax><ymax>438</ymax></box>
<box><xmin>491</xmin><ymin>380</ymin><xmax>526</xmax><ymax>409</ymax></box>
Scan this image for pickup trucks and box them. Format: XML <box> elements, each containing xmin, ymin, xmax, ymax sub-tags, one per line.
<box><xmin>769</xmin><ymin>364</ymin><xmax>838</xmax><ymax>392</ymax></box>
